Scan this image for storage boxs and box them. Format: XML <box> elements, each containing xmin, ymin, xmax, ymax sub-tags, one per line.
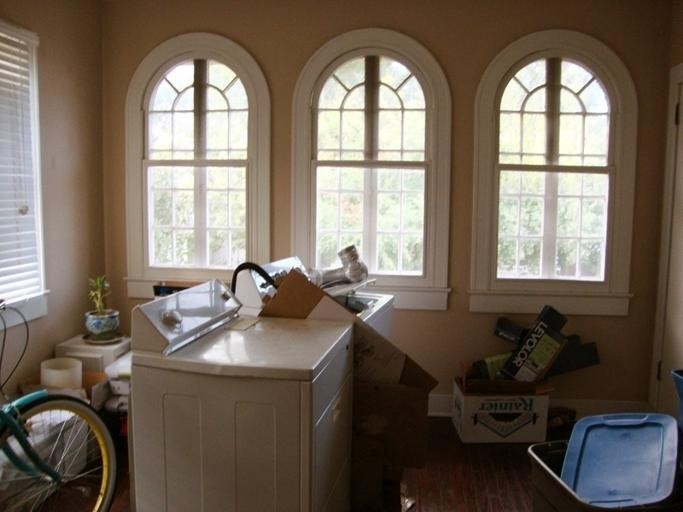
<box><xmin>19</xmin><ymin>370</ymin><xmax>109</xmax><ymax>465</ymax></box>
<box><xmin>524</xmin><ymin>436</ymin><xmax>682</xmax><ymax>512</ymax></box>
<box><xmin>53</xmin><ymin>333</ymin><xmax>133</xmax><ymax>386</ymax></box>
<box><xmin>449</xmin><ymin>375</ymin><xmax>550</xmax><ymax>447</ymax></box>
<box><xmin>0</xmin><ymin>432</ymin><xmax>67</xmax><ymax>512</ymax></box>
<box><xmin>24</xmin><ymin>407</ymin><xmax>90</xmax><ymax>485</ymax></box>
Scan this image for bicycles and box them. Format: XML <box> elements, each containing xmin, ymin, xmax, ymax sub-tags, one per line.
<box><xmin>0</xmin><ymin>298</ymin><xmax>121</xmax><ymax>511</ymax></box>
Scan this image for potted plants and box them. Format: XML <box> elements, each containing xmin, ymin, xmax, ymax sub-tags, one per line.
<box><xmin>82</xmin><ymin>273</ymin><xmax>123</xmax><ymax>347</ymax></box>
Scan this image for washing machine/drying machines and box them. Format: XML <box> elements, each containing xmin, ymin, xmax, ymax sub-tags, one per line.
<box><xmin>126</xmin><ymin>278</ymin><xmax>355</xmax><ymax>511</ymax></box>
<box><xmin>251</xmin><ymin>254</ymin><xmax>398</xmax><ymax>345</ymax></box>
